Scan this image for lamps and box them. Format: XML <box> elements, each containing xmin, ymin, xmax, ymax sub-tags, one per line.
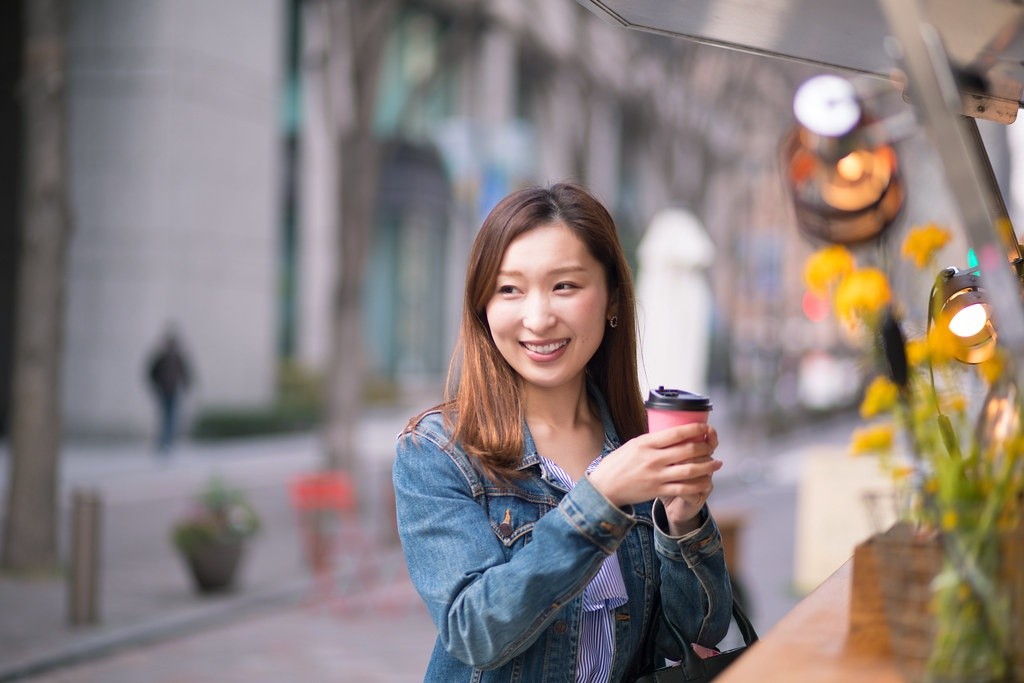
<box><xmin>928</xmin><ymin>264</ymin><xmax>999</xmax><ymax>363</ymax></box>
<box><xmin>775</xmin><ymin>72</ymin><xmax>923</xmax><ymax>246</ymax></box>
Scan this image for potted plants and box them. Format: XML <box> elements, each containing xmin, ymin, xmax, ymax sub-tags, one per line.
<box><xmin>171</xmin><ymin>479</ymin><xmax>258</xmax><ymax>591</ymax></box>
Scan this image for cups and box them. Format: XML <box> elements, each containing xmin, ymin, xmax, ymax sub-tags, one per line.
<box><xmin>644</xmin><ymin>385</ymin><xmax>713</xmax><ymax>441</ymax></box>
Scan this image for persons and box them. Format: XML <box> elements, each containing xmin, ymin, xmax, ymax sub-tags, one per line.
<box><xmin>392</xmin><ymin>183</ymin><xmax>733</xmax><ymax>683</ymax></box>
